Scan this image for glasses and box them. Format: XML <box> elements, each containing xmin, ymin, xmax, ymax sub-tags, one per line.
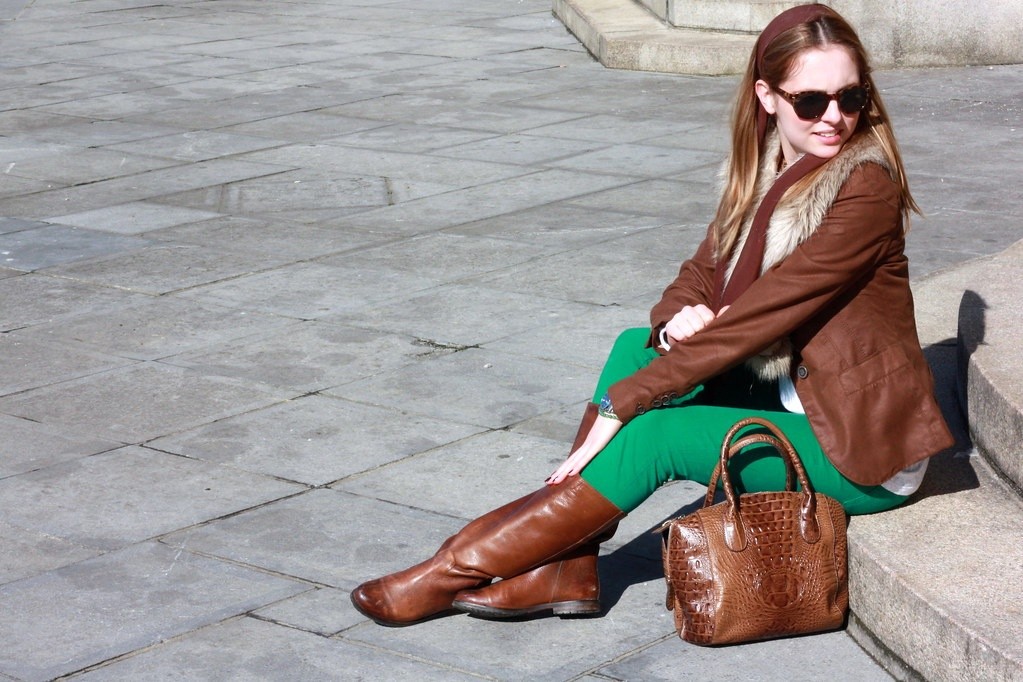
<box><xmin>770</xmin><ymin>78</ymin><xmax>871</xmax><ymax>120</ymax></box>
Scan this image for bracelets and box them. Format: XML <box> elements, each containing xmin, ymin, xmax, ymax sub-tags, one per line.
<box><xmin>658</xmin><ymin>327</ymin><xmax>672</xmax><ymax>351</ymax></box>
<box><xmin>598</xmin><ymin>406</ymin><xmax>619</xmax><ymax>419</ymax></box>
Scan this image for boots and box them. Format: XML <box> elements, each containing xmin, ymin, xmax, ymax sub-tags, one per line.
<box><xmin>350</xmin><ymin>472</ymin><xmax>629</xmax><ymax>626</ymax></box>
<box><xmin>451</xmin><ymin>401</ymin><xmax>619</xmax><ymax>617</ymax></box>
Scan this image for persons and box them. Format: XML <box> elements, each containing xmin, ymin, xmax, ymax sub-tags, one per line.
<box><xmin>350</xmin><ymin>2</ymin><xmax>956</xmax><ymax>630</ymax></box>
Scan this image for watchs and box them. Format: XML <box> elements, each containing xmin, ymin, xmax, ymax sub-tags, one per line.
<box><xmin>600</xmin><ymin>392</ymin><xmax>615</xmax><ymax>413</ymax></box>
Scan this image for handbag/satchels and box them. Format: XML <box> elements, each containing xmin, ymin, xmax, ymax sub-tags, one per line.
<box><xmin>650</xmin><ymin>418</ymin><xmax>849</xmax><ymax>646</ymax></box>
